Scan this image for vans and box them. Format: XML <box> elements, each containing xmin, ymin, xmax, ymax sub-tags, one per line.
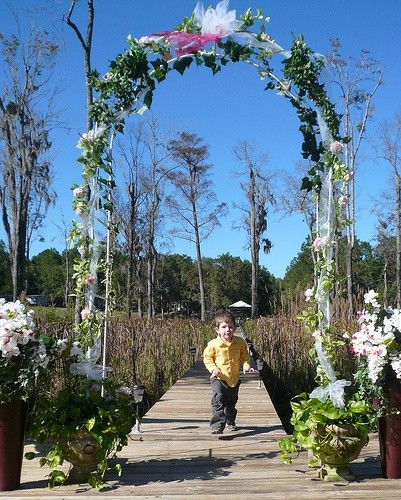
<box><xmin>27</xmin><ymin>295</ymin><xmax>52</xmax><ymax>307</ymax></box>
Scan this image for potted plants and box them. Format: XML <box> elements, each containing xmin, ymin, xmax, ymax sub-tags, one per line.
<box><xmin>277</xmin><ymin>391</ymin><xmax>376</xmax><ymax>481</ymax></box>
<box><xmin>23</xmin><ymin>377</ymin><xmax>142</xmax><ymax>488</ymax></box>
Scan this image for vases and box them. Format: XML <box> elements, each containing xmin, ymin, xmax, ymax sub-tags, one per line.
<box><xmin>379</xmin><ymin>384</ymin><xmax>401</xmax><ymax>479</ymax></box>
<box><xmin>0</xmin><ymin>400</ymin><xmax>27</xmax><ymax>491</ymax></box>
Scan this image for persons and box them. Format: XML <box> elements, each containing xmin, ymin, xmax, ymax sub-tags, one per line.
<box><xmin>203</xmin><ymin>311</ymin><xmax>255</xmax><ymax>434</ymax></box>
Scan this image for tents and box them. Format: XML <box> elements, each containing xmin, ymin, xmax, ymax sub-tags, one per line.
<box><xmin>228</xmin><ymin>300</ymin><xmax>252</xmax><ymax>326</ymax></box>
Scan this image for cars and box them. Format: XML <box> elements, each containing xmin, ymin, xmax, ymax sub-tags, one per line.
<box><xmin>176</xmin><ymin>302</ymin><xmax>193</xmax><ymax>313</ymax></box>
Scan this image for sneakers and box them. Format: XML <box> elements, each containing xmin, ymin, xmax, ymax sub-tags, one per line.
<box><xmin>211</xmin><ymin>427</ymin><xmax>223</xmax><ymax>434</ymax></box>
<box><xmin>227</xmin><ymin>425</ymin><xmax>237</xmax><ymax>431</ymax></box>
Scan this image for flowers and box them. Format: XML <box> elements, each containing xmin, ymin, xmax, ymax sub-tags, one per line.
<box><xmin>139</xmin><ymin>36</ymin><xmax>153</xmax><ymax>46</ymax></box>
<box><xmin>342</xmin><ymin>289</ymin><xmax>401</xmax><ymax>417</ymax></box>
<box><xmin>70</xmin><ymin>130</ymin><xmax>113</xmax><ymax>376</ymax></box>
<box><xmin>305</xmin><ymin>140</ymin><xmax>355</xmax><ymax>391</ymax></box>
<box><xmin>0</xmin><ymin>299</ymin><xmax>69</xmax><ymax>406</ymax></box>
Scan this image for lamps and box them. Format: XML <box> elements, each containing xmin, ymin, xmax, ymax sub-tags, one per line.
<box><xmin>255</xmin><ymin>358</ymin><xmax>264</xmax><ymax>371</ymax></box>
<box><xmin>132</xmin><ymin>385</ymin><xmax>147</xmax><ymax>403</ymax></box>
<box><xmin>190</xmin><ymin>348</ymin><xmax>197</xmax><ymax>356</ymax></box>
<box><xmin>247</xmin><ymin>340</ymin><xmax>252</xmax><ymax>348</ymax></box>
